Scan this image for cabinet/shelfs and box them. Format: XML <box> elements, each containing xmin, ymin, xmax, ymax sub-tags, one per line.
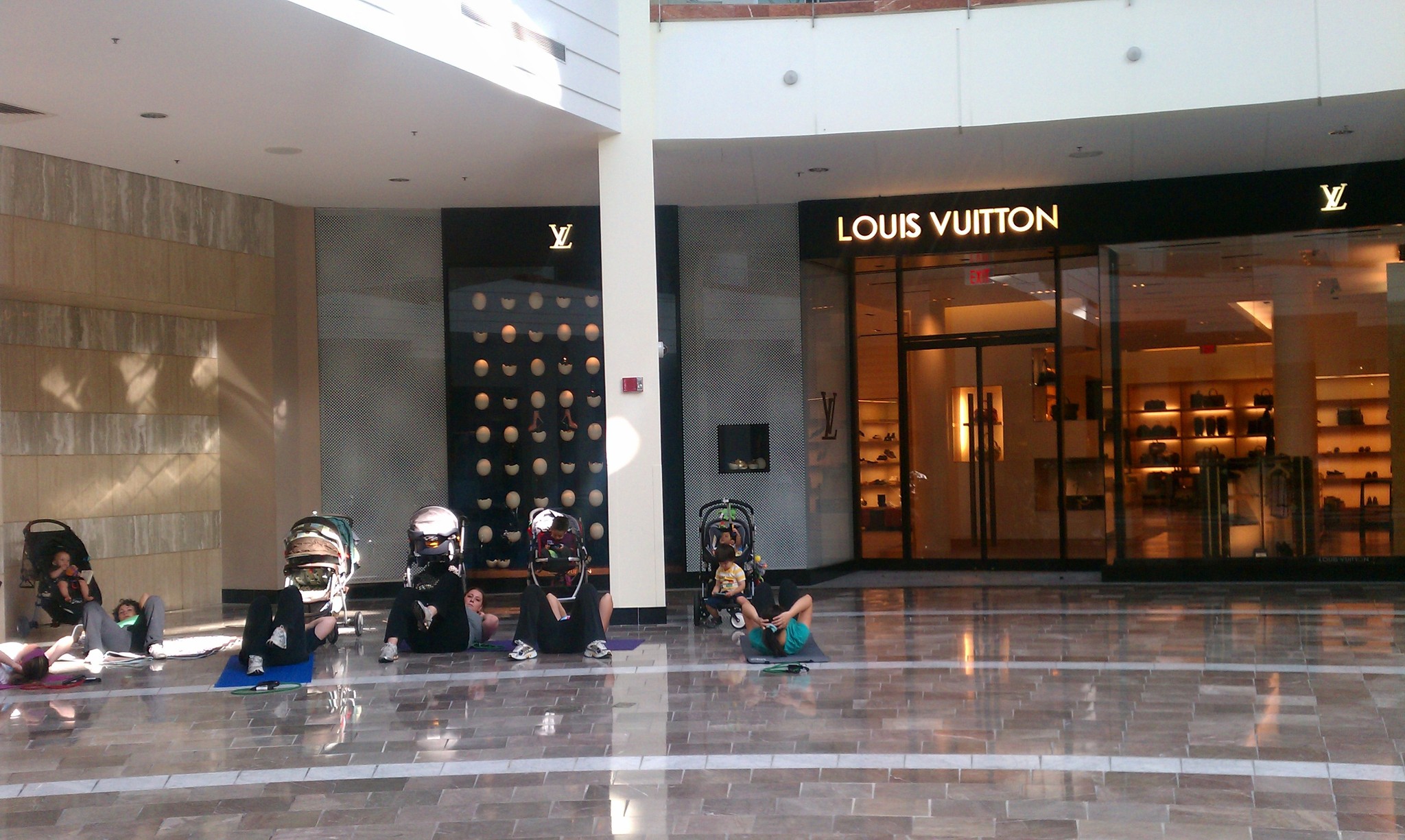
<box><xmin>1025</xmin><ymin>343</ymin><xmax>1103</xmax><ymax>520</ymax></box>
<box><xmin>856</xmin><ymin>396</ymin><xmax>909</xmax><ymax>530</ymax></box>
<box><xmin>1312</xmin><ymin>371</ymin><xmax>1396</xmax><ymax>551</ymax></box>
<box><xmin>946</xmin><ymin>383</ymin><xmax>1007</xmax><ymax>471</ymax></box>
<box><xmin>1099</xmin><ymin>342</ymin><xmax>1276</xmax><ymax>482</ymax></box>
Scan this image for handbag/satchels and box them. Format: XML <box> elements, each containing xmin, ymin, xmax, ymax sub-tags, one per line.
<box><xmin>1143</xmin><ymin>399</ymin><xmax>1167</xmax><ymax>409</ymax></box>
<box><xmin>976</xmin><ymin>432</ymin><xmax>1001</xmax><ymax>461</ymax></box>
<box><xmin>1253</xmin><ymin>388</ymin><xmax>1274</xmax><ymax>406</ymax></box>
<box><xmin>1050</xmin><ymin>396</ymin><xmax>1080</xmax><ymax>421</ymax></box>
<box><xmin>1190</xmin><ymin>388</ymin><xmax>1228</xmax><ymax>409</ymax></box>
<box><xmin>1336</xmin><ymin>406</ymin><xmax>1365</xmax><ymax>426</ymax></box>
<box><xmin>974</xmin><ymin>400</ymin><xmax>998</xmax><ymax>423</ymax></box>
<box><xmin>1137</xmin><ymin>415</ymin><xmax>1267</xmax><ymax>502</ymax></box>
<box><xmin>1036</xmin><ymin>360</ymin><xmax>1055</xmax><ymax>385</ymax></box>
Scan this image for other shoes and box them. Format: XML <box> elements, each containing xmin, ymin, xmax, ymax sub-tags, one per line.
<box><xmin>859</xmin><ymin>429</ymin><xmax>896</xmax><ymax>484</ymax></box>
<box><xmin>1275</xmin><ymin>542</ymin><xmax>1293</xmax><ymax>560</ymax></box>
<box><xmin>1324</xmin><ymin>446</ymin><xmax>1380</xmax><ymax>508</ymax></box>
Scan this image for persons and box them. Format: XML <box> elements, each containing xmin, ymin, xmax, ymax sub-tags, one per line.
<box><xmin>540</xmin><ymin>516</ymin><xmax>574</xmax><ymax>570</ymax></box>
<box><xmin>82</xmin><ymin>593</ymin><xmax>169</xmax><ymax>674</ymax></box>
<box><xmin>741</xmin><ymin>579</ymin><xmax>813</xmax><ymax>659</ymax></box>
<box><xmin>378</xmin><ymin>572</ymin><xmax>499</xmax><ymax>663</ymax></box>
<box><xmin>705</xmin><ymin>543</ymin><xmax>747</xmax><ymax>628</ymax></box>
<box><xmin>238</xmin><ymin>584</ymin><xmax>337</xmax><ymax>674</ymax></box>
<box><xmin>0</xmin><ymin>636</ymin><xmax>74</xmax><ymax>686</ymax></box>
<box><xmin>712</xmin><ymin>527</ymin><xmax>742</xmax><ymax>558</ymax></box>
<box><xmin>48</xmin><ymin>549</ymin><xmax>94</xmax><ymax>602</ymax></box>
<box><xmin>509</xmin><ymin>583</ymin><xmax>613</xmax><ymax>660</ymax></box>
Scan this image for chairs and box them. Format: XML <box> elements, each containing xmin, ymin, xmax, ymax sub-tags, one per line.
<box><xmin>1358</xmin><ymin>477</ymin><xmax>1395</xmax><ymax>538</ymax></box>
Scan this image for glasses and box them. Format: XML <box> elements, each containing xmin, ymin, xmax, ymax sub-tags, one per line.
<box><xmin>62</xmin><ymin>674</ymin><xmax>87</xmax><ymax>685</ymax></box>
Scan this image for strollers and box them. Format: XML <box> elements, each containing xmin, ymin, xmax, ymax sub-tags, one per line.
<box><xmin>401</xmin><ymin>503</ymin><xmax>468</xmax><ymax>596</ymax></box>
<box><xmin>14</xmin><ymin>518</ymin><xmax>103</xmax><ymax>639</ymax></box>
<box><xmin>692</xmin><ymin>498</ymin><xmax>764</xmax><ymax>630</ymax></box>
<box><xmin>526</xmin><ymin>506</ymin><xmax>589</xmax><ymax>604</ymax></box>
<box><xmin>283</xmin><ymin>509</ymin><xmax>365</xmax><ymax>645</ymax></box>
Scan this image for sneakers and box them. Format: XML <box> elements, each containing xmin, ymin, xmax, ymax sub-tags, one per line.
<box><xmin>508</xmin><ymin>639</ymin><xmax>538</xmax><ymax>660</ymax></box>
<box><xmin>84</xmin><ymin>648</ymin><xmax>104</xmax><ymax>663</ymax></box>
<box><xmin>266</xmin><ymin>624</ymin><xmax>287</xmax><ymax>649</ymax></box>
<box><xmin>413</xmin><ymin>599</ymin><xmax>433</xmax><ymax>633</ymax></box>
<box><xmin>149</xmin><ymin>643</ymin><xmax>166</xmax><ymax>660</ymax></box>
<box><xmin>584</xmin><ymin>640</ymin><xmax>612</xmax><ymax>659</ymax></box>
<box><xmin>378</xmin><ymin>642</ymin><xmax>399</xmax><ymax>663</ymax></box>
<box><xmin>247</xmin><ymin>654</ymin><xmax>265</xmax><ymax>675</ymax></box>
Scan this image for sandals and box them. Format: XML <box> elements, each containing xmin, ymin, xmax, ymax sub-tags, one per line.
<box><xmin>707</xmin><ymin>616</ymin><xmax>722</xmax><ymax>628</ymax></box>
